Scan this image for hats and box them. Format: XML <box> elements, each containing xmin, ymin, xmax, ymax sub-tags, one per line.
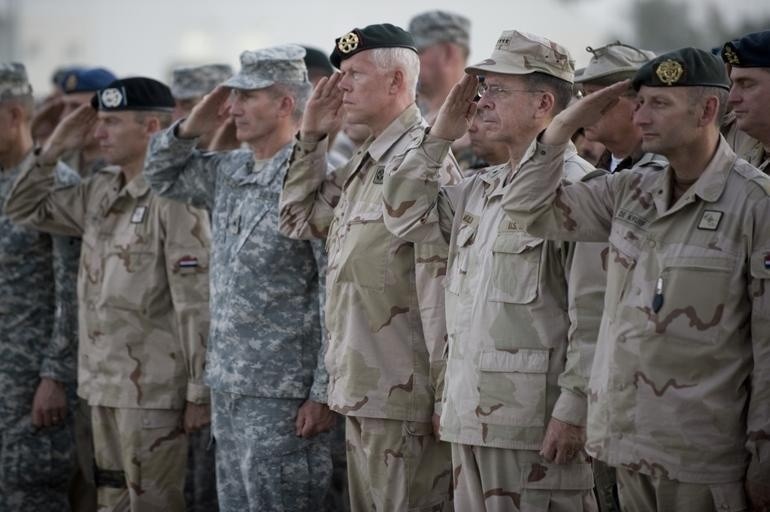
<box><xmin>90</xmin><ymin>77</ymin><xmax>176</xmax><ymax>114</ymax></box>
<box><xmin>0</xmin><ymin>61</ymin><xmax>32</xmax><ymax>97</ymax></box>
<box><xmin>217</xmin><ymin>46</ymin><xmax>309</xmax><ymax>90</ymax></box>
<box><xmin>409</xmin><ymin>10</ymin><xmax>471</xmax><ymax>53</ymax></box>
<box><xmin>465</xmin><ymin>27</ymin><xmax>577</xmax><ymax>84</ymax></box>
<box><xmin>721</xmin><ymin>29</ymin><xmax>770</xmax><ymax>70</ymax></box>
<box><xmin>59</xmin><ymin>66</ymin><xmax>116</xmax><ymax>93</ymax></box>
<box><xmin>329</xmin><ymin>23</ymin><xmax>419</xmax><ymax>70</ymax></box>
<box><xmin>171</xmin><ymin>62</ymin><xmax>233</xmax><ymax>100</ymax></box>
<box><xmin>575</xmin><ymin>41</ymin><xmax>656</xmax><ymax>83</ymax></box>
<box><xmin>631</xmin><ymin>45</ymin><xmax>732</xmax><ymax>93</ymax></box>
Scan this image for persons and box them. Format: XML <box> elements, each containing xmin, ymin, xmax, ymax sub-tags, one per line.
<box><xmin>380</xmin><ymin>29</ymin><xmax>610</xmax><ymax>511</ymax></box>
<box><xmin>450</xmin><ymin>95</ymin><xmax>510</xmax><ymax>177</ymax></box>
<box><xmin>276</xmin><ymin>24</ymin><xmax>464</xmax><ymax>511</ymax></box>
<box><xmin>0</xmin><ymin>60</ymin><xmax>84</xmax><ymax>512</ymax></box>
<box><xmin>1</xmin><ymin>76</ymin><xmax>212</xmax><ymax>512</ymax></box>
<box><xmin>142</xmin><ymin>44</ymin><xmax>339</xmax><ymax>511</ymax></box>
<box><xmin>573</xmin><ymin>40</ymin><xmax>668</xmax><ymax>512</ymax></box>
<box><xmin>721</xmin><ymin>30</ymin><xmax>770</xmax><ymax>175</ymax></box>
<box><xmin>500</xmin><ymin>46</ymin><xmax>769</xmax><ymax>512</ymax></box>
<box><xmin>170</xmin><ymin>63</ymin><xmax>241</xmax><ymax>512</ymax></box>
<box><xmin>31</xmin><ymin>67</ymin><xmax>119</xmax><ymax>511</ymax></box>
<box><xmin>408</xmin><ymin>10</ymin><xmax>489</xmax><ymax>172</ymax></box>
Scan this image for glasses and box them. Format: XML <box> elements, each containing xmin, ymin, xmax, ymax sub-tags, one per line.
<box><xmin>477</xmin><ymin>84</ymin><xmax>545</xmax><ymax>98</ymax></box>
